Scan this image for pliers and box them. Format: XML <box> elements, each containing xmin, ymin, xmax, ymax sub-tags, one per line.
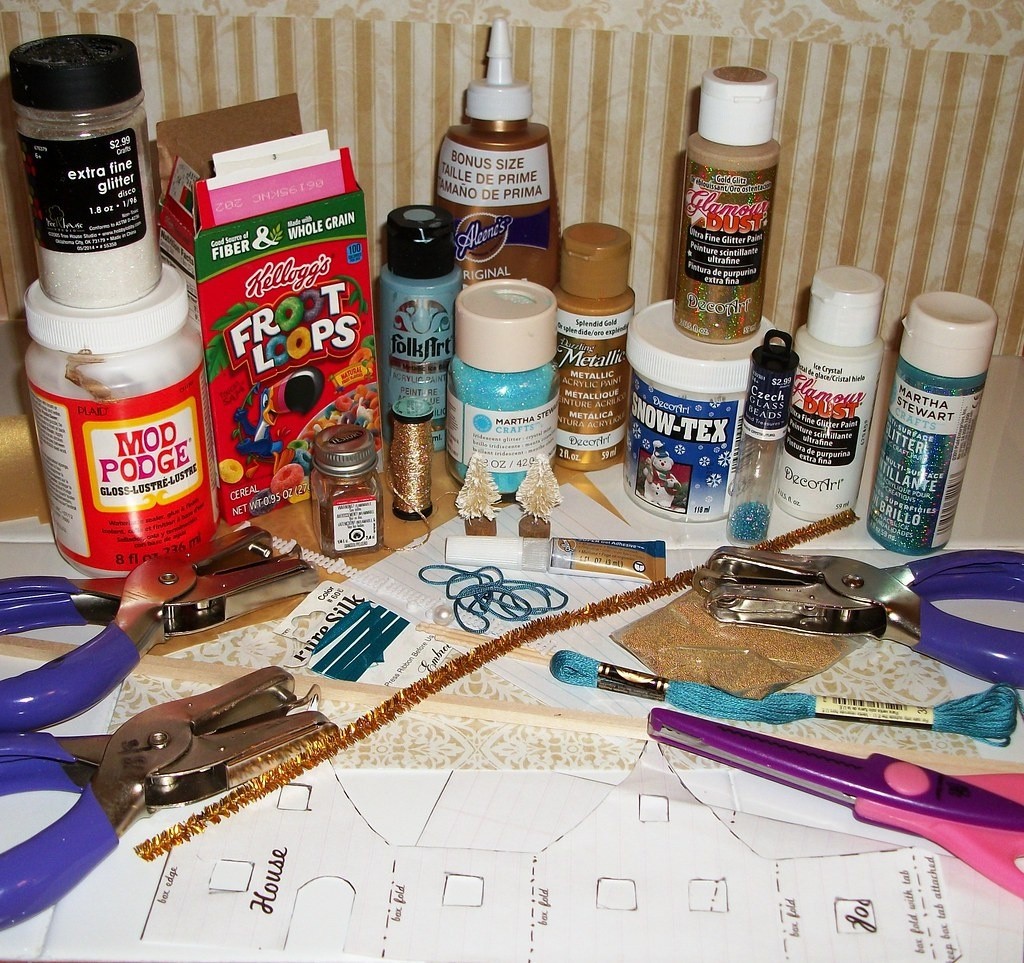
<box><xmin>705</xmin><ymin>544</ymin><xmax>1024</xmax><ymax>686</ymax></box>
<box><xmin>0</xmin><ymin>661</ymin><xmax>337</xmax><ymax>930</ymax></box>
<box><xmin>1</xmin><ymin>526</ymin><xmax>313</xmax><ymax>732</ymax></box>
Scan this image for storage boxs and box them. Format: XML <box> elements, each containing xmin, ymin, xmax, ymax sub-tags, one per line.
<box><xmin>155</xmin><ymin>92</ymin><xmax>378</xmax><ymax>529</ymax></box>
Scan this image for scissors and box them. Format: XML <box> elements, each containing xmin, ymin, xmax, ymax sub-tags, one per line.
<box><xmin>643</xmin><ymin>706</ymin><xmax>1023</xmax><ymax>898</ymax></box>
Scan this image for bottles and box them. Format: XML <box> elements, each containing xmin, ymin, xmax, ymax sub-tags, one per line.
<box><xmin>774</xmin><ymin>265</ymin><xmax>885</xmax><ymax>523</ymax></box>
<box><xmin>9</xmin><ymin>34</ymin><xmax>163</xmax><ymax>309</ymax></box>
<box><xmin>436</xmin><ymin>18</ymin><xmax>563</xmax><ymax>294</ymax></box>
<box><xmin>24</xmin><ymin>262</ymin><xmax>220</xmax><ymax>582</ymax></box>
<box><xmin>379</xmin><ymin>205</ymin><xmax>463</xmax><ymax>452</ymax></box>
<box><xmin>554</xmin><ymin>222</ymin><xmax>635</xmax><ymax>471</ymax></box>
<box><xmin>309</xmin><ymin>425</ymin><xmax>385</xmax><ymax>558</ymax></box>
<box><xmin>671</xmin><ymin>66</ymin><xmax>780</xmax><ymax>345</ymax></box>
<box><xmin>866</xmin><ymin>291</ymin><xmax>998</xmax><ymax>556</ymax></box>
<box><xmin>447</xmin><ymin>279</ymin><xmax>559</xmax><ymax>494</ymax></box>
